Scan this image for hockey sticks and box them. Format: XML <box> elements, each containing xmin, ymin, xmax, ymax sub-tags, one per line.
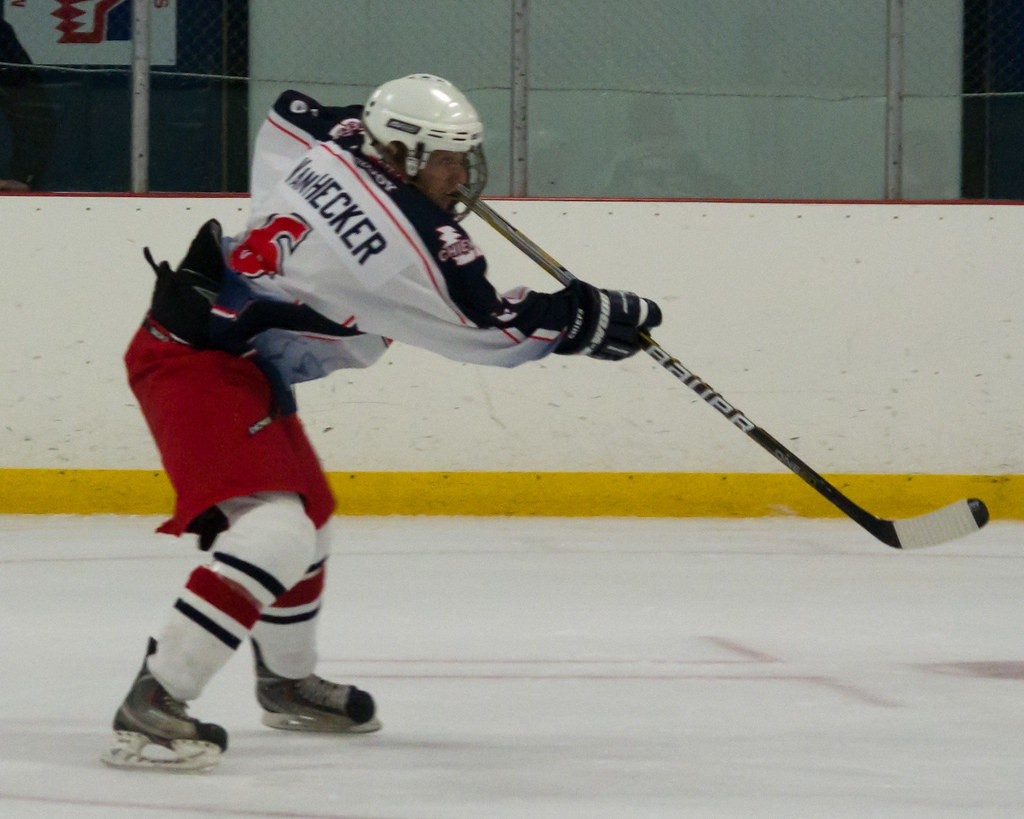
<box><xmin>451</xmin><ymin>180</ymin><xmax>993</xmax><ymax>551</ymax></box>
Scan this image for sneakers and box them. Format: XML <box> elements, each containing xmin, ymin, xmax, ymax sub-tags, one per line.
<box><xmin>251</xmin><ymin>658</ymin><xmax>384</xmax><ymax>734</ymax></box>
<box><xmin>100</xmin><ymin>656</ymin><xmax>228</xmax><ymax>771</ymax></box>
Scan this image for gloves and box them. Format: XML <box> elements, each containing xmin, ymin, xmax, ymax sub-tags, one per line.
<box><xmin>545</xmin><ymin>278</ymin><xmax>662</xmax><ymax>360</ymax></box>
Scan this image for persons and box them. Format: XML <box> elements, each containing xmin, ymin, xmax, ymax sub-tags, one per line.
<box><xmin>110</xmin><ymin>71</ymin><xmax>665</xmax><ymax>756</ymax></box>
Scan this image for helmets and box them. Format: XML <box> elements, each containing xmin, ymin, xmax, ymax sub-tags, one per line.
<box><xmin>357</xmin><ymin>70</ymin><xmax>488</xmax><ymax>223</ymax></box>
